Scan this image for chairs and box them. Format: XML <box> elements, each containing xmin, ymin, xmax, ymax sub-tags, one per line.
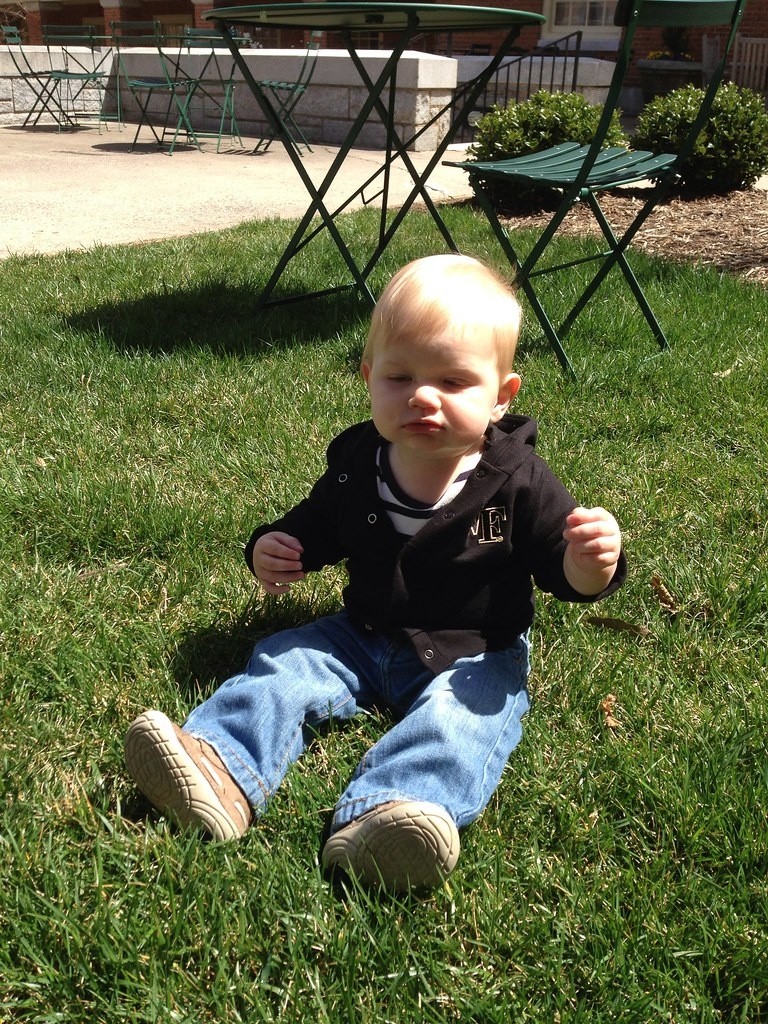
<box><xmin>1</xmin><ymin>20</ymin><xmax>322</xmax><ymax>154</ymax></box>
<box><xmin>440</xmin><ymin>0</ymin><xmax>746</xmax><ymax>385</ymax></box>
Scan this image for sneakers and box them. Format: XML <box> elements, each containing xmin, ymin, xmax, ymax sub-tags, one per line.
<box><xmin>322</xmin><ymin>801</ymin><xmax>460</xmax><ymax>896</ymax></box>
<box><xmin>126</xmin><ymin>709</ymin><xmax>252</xmax><ymax>845</ymax></box>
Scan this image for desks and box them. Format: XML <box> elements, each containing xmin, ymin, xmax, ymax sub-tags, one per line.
<box><xmin>201</xmin><ymin>1</ymin><xmax>548</xmax><ymax>306</ymax></box>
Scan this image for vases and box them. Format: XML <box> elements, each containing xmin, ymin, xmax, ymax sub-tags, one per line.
<box><xmin>635</xmin><ymin>58</ymin><xmax>702</xmax><ymax>106</ymax></box>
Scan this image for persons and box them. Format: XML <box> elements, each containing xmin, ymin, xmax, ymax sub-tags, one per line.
<box><xmin>125</xmin><ymin>253</ymin><xmax>627</xmax><ymax>899</ymax></box>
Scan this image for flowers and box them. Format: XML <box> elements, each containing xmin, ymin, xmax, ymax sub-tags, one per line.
<box><xmin>646</xmin><ymin>26</ymin><xmax>696</xmax><ymax>62</ymax></box>
